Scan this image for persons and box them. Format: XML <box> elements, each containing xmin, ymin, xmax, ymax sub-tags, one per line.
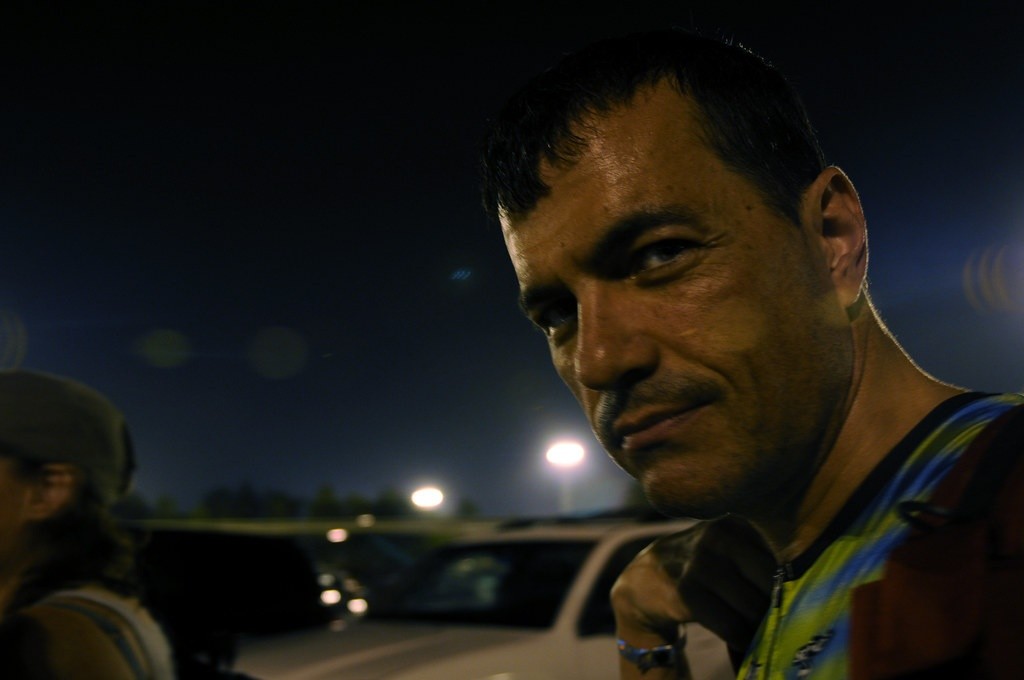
<box><xmin>480</xmin><ymin>31</ymin><xmax>1024</xmax><ymax>680</ymax></box>
<box><xmin>0</xmin><ymin>369</ymin><xmax>174</xmax><ymax>680</ymax></box>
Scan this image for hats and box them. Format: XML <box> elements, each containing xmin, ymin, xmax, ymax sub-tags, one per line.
<box><xmin>0</xmin><ymin>369</ymin><xmax>136</xmax><ymax>493</ymax></box>
